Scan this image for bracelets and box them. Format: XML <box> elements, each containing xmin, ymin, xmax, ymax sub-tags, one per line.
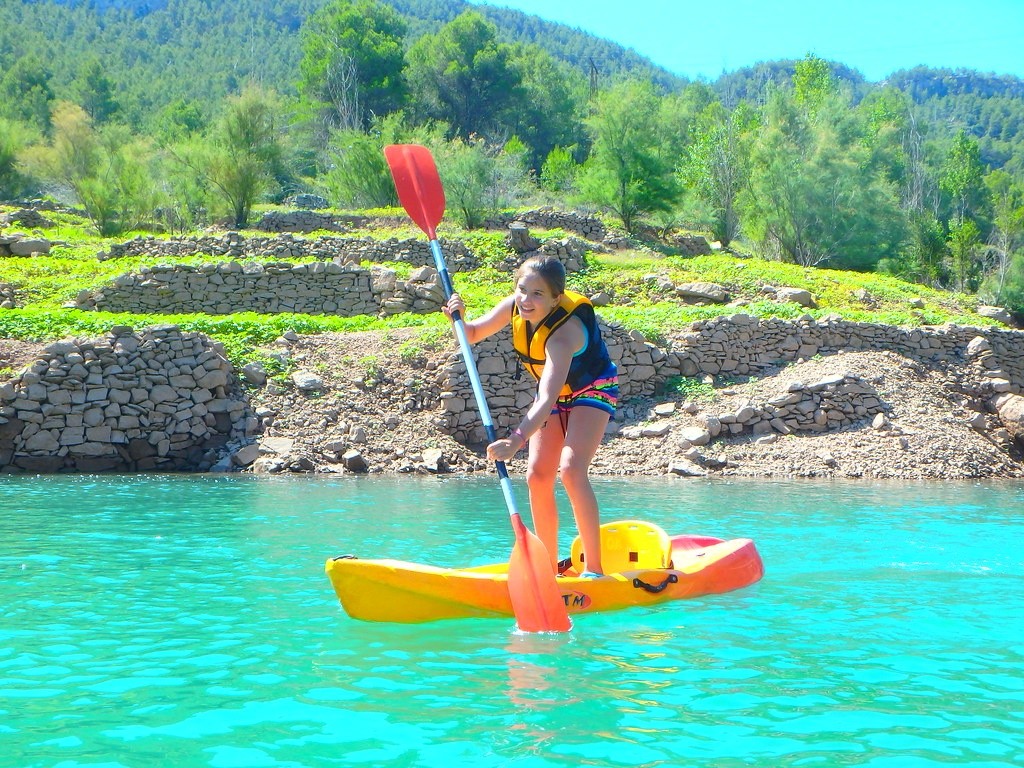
<box><xmin>511</xmin><ymin>430</ymin><xmax>528</xmax><ymax>444</ymax></box>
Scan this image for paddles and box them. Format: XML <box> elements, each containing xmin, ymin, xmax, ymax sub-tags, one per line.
<box><xmin>380</xmin><ymin>137</ymin><xmax>574</xmax><ymax>634</ymax></box>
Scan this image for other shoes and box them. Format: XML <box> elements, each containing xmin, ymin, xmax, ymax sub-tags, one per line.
<box><xmin>580</xmin><ymin>572</ymin><xmax>604</xmax><ymax>578</ymax></box>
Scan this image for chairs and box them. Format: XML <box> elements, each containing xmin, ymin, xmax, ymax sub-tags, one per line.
<box><xmin>570</xmin><ymin>519</ymin><xmax>673</xmax><ymax>574</ymax></box>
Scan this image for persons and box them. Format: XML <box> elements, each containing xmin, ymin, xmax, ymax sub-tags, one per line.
<box><xmin>442</xmin><ymin>254</ymin><xmax>619</xmax><ymax>579</ymax></box>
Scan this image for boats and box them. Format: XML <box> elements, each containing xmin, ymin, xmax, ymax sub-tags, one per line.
<box><xmin>324</xmin><ymin>519</ymin><xmax>765</xmax><ymax>624</ymax></box>
